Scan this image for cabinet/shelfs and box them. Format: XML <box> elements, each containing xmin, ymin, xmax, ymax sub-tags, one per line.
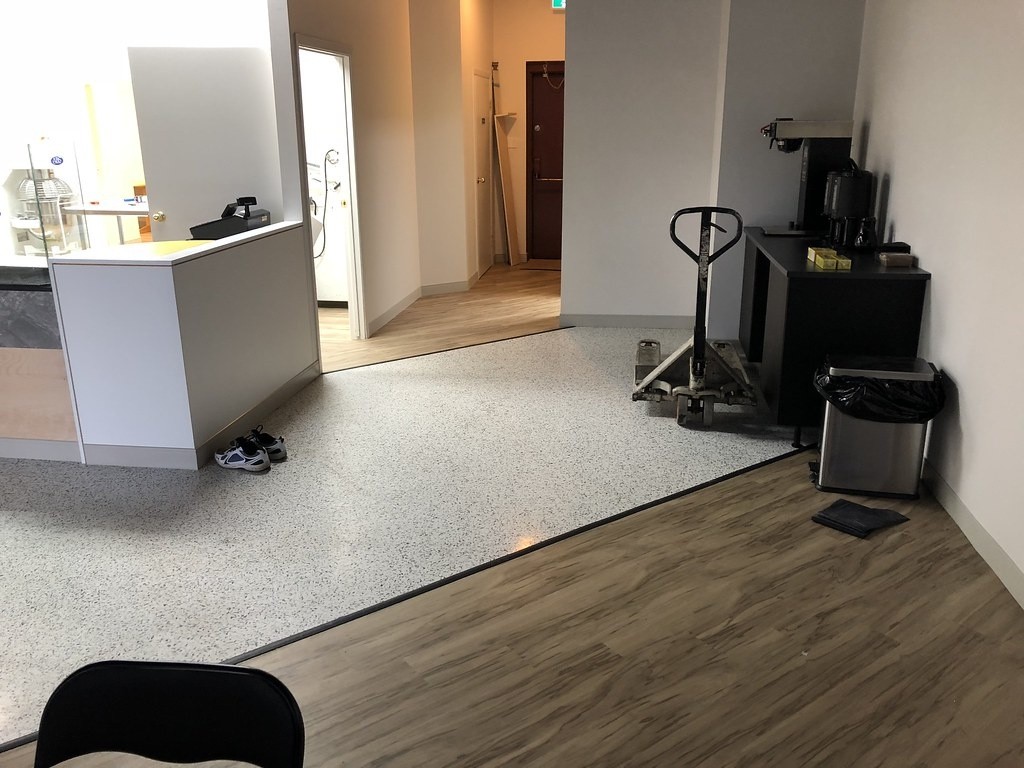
<box><xmin>738</xmin><ymin>225</ymin><xmax>932</xmax><ymax>449</ymax></box>
<box><xmin>0</xmin><ymin>266</ymin><xmax>85</xmax><ymax>463</ymax></box>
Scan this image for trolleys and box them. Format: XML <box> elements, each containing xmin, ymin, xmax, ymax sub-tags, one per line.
<box><xmin>633</xmin><ymin>205</ymin><xmax>758</xmax><ymax>428</ymax></box>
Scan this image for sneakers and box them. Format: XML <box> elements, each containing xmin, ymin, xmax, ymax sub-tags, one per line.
<box><xmin>214</xmin><ymin>437</ymin><xmax>271</xmax><ymax>471</ymax></box>
<box><xmin>229</xmin><ymin>425</ymin><xmax>286</xmax><ymax>461</ymax></box>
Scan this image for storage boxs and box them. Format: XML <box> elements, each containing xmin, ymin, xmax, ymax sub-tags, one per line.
<box><xmin>807</xmin><ymin>247</ymin><xmax>851</xmax><ymax>270</ymax></box>
<box><xmin>878</xmin><ymin>253</ymin><xmax>914</xmax><ymax>268</ymax></box>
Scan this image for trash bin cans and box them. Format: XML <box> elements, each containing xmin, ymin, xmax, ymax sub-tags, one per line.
<box><xmin>812</xmin><ymin>350</ymin><xmax>936</xmax><ymax>500</ymax></box>
